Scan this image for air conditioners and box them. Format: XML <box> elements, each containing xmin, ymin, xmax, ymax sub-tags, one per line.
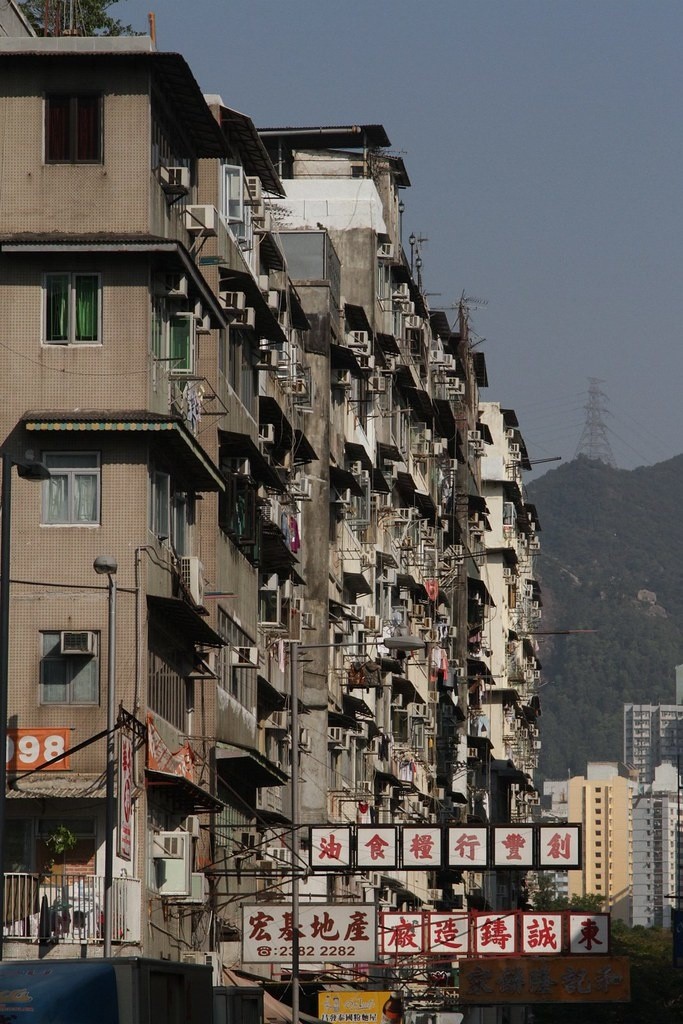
<box><xmin>234</xmin><ymin>307</ymin><xmax>256</xmax><ymax>331</ymax></box>
<box><xmin>232</xmin><ymin>646</ymin><xmax>257</xmax><ymax>665</ymax></box>
<box><xmin>234</xmin><ymin>458</ymin><xmax>252</xmax><ymax>475</ymax></box>
<box><xmin>60</xmin><ymin>631</ymin><xmax>94</xmax><ymax>655</ymax></box>
<box><xmin>163</xmin><ymin>836</ymin><xmax>184</xmax><ymax>857</ymax></box>
<box><xmin>161</xmin><ymin>167</ymin><xmax>190</xmax><ymax>191</ymax></box>
<box><xmin>179</xmin><ymin>814</ymin><xmax>199</xmax><ymax>835</ymax></box>
<box><xmin>154</xmin><ymin>268</ymin><xmax>211</xmax><ymax>336</ymax></box>
<box><xmin>247</xmin><ymin>176</ymin><xmax>275</xmax><ymax>233</ymax></box>
<box><xmin>430</xmin><ymin>889</ymin><xmax>443</xmax><ymax>899</ymax></box>
<box><xmin>185</xmin><ymin>204</ymin><xmax>218</xmax><ymax>236</ymax></box>
<box><xmin>179</xmin><ymin>557</ymin><xmax>204</xmax><ymax>606</ymax></box>
<box><xmin>258</xmin><ymin>242</ymin><xmax>541</xmax><ymax>864</ymax></box>
<box><xmin>179</xmin><ymin>949</ymin><xmax>218</xmax><ymax>972</ymax></box>
<box><xmin>225</xmin><ymin>291</ymin><xmax>247</xmax><ymax>313</ymax></box>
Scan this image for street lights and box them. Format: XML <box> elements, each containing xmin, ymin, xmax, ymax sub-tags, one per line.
<box><xmin>91</xmin><ymin>554</ymin><xmax>116</xmax><ymax>959</ymax></box>
<box><xmin>286</xmin><ymin>634</ymin><xmax>428</xmax><ymax>1024</ymax></box>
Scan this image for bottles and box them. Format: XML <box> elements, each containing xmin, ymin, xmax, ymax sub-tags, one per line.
<box><xmin>380</xmin><ymin>991</ymin><xmax>403</xmax><ymax>1024</ymax></box>
